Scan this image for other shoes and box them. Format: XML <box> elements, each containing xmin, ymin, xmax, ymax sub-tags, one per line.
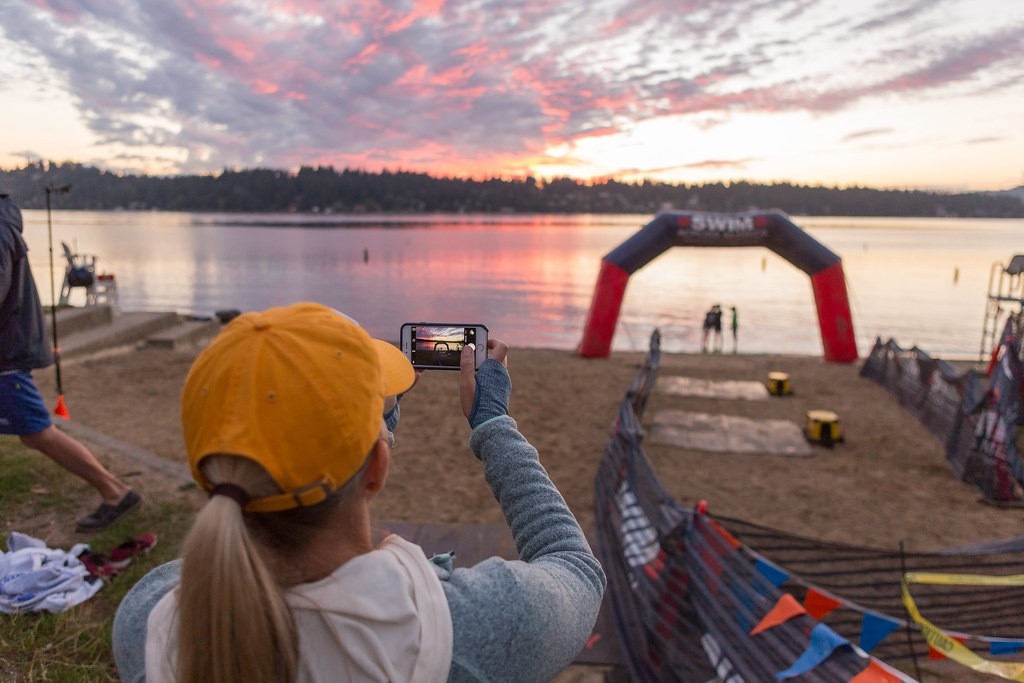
<box><xmin>108</xmin><ymin>534</ymin><xmax>157</xmax><ymax>571</ymax></box>
<box><xmin>76</xmin><ymin>488</ymin><xmax>141</xmax><ymax>534</ymax></box>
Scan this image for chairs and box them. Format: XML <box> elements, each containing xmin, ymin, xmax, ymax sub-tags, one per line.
<box><xmin>86</xmin><ymin>272</ymin><xmax>119</xmax><ymax>320</ymax></box>
<box><xmin>59</xmin><ymin>241</ymin><xmax>98</xmax><ymax>307</ymax></box>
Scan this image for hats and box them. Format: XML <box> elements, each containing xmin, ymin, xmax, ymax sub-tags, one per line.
<box><xmin>182</xmin><ymin>302</ymin><xmax>416</xmax><ymax>512</ymax></box>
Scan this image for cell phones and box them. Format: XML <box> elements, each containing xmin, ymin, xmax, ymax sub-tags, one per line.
<box><xmin>400</xmin><ymin>321</ymin><xmax>488</xmax><ymax>370</ymax></box>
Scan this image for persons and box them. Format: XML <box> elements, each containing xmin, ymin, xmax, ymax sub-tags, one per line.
<box><xmin>0</xmin><ymin>194</ymin><xmax>142</xmax><ymax>532</ymax></box>
<box><xmin>111</xmin><ymin>301</ymin><xmax>608</xmax><ymax>683</ymax></box>
<box><xmin>702</xmin><ymin>305</ymin><xmax>738</xmax><ymax>355</ymax></box>
